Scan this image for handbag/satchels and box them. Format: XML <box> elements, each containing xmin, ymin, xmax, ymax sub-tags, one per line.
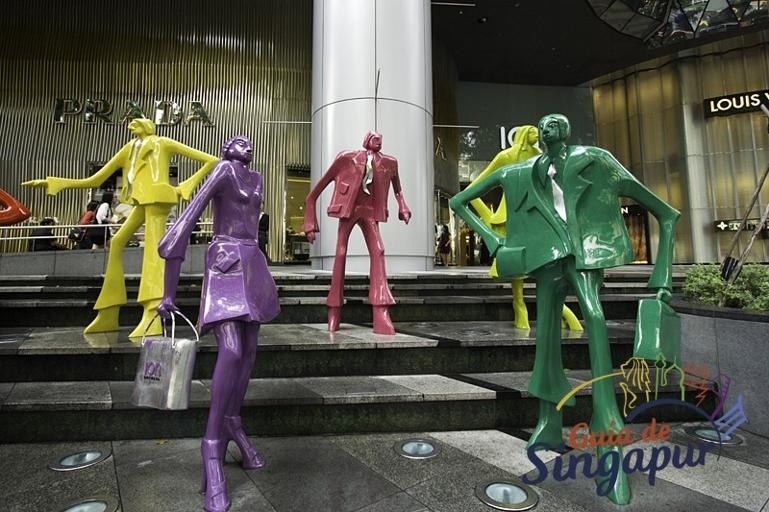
<box><xmin>86</xmin><ymin>228</ymin><xmax>105</xmax><ymax>246</ymax></box>
<box><xmin>68</xmin><ymin>229</ymin><xmax>86</xmax><ymax>242</ymax></box>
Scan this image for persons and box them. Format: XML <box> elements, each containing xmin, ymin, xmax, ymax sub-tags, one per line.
<box><xmin>257</xmin><ymin>199</ymin><xmax>274</xmax><ymax>265</ymax></box>
<box><xmin>26</xmin><ymin>190</ymin><xmax>205</xmax><ymax>252</ymax></box>
<box><xmin>156</xmin><ymin>132</ymin><xmax>283</xmax><ymax>512</ymax></box>
<box><xmin>478</xmin><ymin>236</ymin><xmax>491</xmax><ymax>266</ymax></box>
<box><xmin>439</xmin><ymin>225</ymin><xmax>452</xmax><ymax>267</ymax></box>
<box><xmin>301</xmin><ymin>128</ymin><xmax>414</xmax><ymax>336</ymax></box>
<box><xmin>462</xmin><ymin>124</ymin><xmax>586</xmax><ymax>333</ymax></box>
<box><xmin>434</xmin><ymin>224</ymin><xmax>437</xmax><ymax>264</ymax></box>
<box><xmin>448</xmin><ymin>113</ymin><xmax>682</xmax><ymax>509</ymax></box>
<box><xmin>17</xmin><ymin>117</ymin><xmax>224</xmax><ymax>339</ymax></box>
